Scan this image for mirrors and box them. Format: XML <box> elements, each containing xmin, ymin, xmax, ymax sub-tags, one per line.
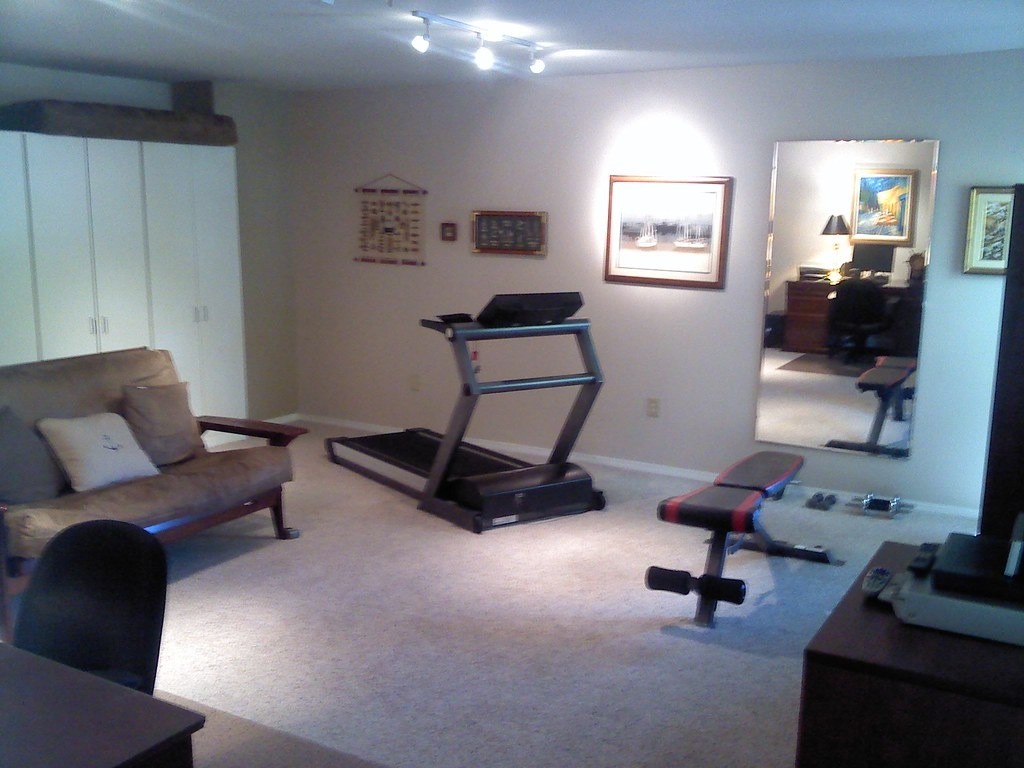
<box><xmin>754</xmin><ymin>140</ymin><xmax>941</xmax><ymax>459</ymax></box>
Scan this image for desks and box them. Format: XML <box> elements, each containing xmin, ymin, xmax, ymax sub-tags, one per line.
<box><xmin>0</xmin><ymin>641</ymin><xmax>206</xmax><ymax>768</ymax></box>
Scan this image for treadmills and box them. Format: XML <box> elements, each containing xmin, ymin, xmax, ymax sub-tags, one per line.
<box><xmin>323</xmin><ymin>291</ymin><xmax>606</xmax><ymax>534</ymax></box>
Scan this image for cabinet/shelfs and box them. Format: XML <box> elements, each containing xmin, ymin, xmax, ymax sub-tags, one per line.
<box><xmin>0</xmin><ymin>129</ymin><xmax>248</xmax><ymax>447</ymax></box>
<box><xmin>783</xmin><ymin>281</ymin><xmax>923</xmax><ymax>357</ymax></box>
<box><xmin>793</xmin><ymin>543</ymin><xmax>1024</xmax><ymax>768</ymax></box>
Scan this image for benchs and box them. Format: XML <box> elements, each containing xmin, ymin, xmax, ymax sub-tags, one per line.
<box><xmin>0</xmin><ymin>347</ymin><xmax>308</xmax><ymax>578</ymax></box>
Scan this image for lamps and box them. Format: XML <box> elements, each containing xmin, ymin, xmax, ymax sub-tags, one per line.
<box><xmin>411</xmin><ymin>11</ymin><xmax>546</xmax><ymax>74</ymax></box>
<box><xmin>819</xmin><ymin>214</ymin><xmax>850</xmax><ymax>281</ymax></box>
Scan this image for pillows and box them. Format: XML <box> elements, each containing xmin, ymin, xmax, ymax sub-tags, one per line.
<box><xmin>36</xmin><ymin>411</ymin><xmax>160</xmax><ymax>493</ymax></box>
<box><xmin>121</xmin><ymin>381</ymin><xmax>202</xmax><ymax>468</ymax></box>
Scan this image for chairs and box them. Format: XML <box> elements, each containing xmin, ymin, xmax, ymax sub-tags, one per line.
<box><xmin>10</xmin><ymin>518</ymin><xmax>167</xmax><ymax>697</ymax></box>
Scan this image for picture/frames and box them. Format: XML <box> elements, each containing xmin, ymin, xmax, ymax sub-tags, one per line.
<box><xmin>472</xmin><ymin>211</ymin><xmax>548</xmax><ymax>256</ymax></box>
<box><xmin>604</xmin><ymin>175</ymin><xmax>733</xmax><ymax>290</ymax></box>
<box><xmin>964</xmin><ymin>186</ymin><xmax>1016</xmax><ymax>275</ymax></box>
<box><xmin>849</xmin><ymin>169</ymin><xmax>919</xmax><ymax>247</ymax></box>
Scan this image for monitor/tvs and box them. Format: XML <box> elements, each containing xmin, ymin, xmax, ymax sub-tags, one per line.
<box><xmin>853</xmin><ymin>243</ymin><xmax>894</xmax><ymax>278</ymax></box>
<box><xmin>934</xmin><ymin>183</ymin><xmax>1024</xmax><ymax>604</ymax></box>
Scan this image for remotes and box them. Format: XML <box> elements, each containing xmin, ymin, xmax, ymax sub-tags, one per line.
<box><xmin>880</xmin><ymin>574</ymin><xmax>908</xmax><ymax>603</ymax></box>
<box><xmin>908</xmin><ymin>543</ymin><xmax>940</xmax><ymax>576</ymax></box>
<box><xmin>862</xmin><ymin>566</ymin><xmax>892</xmax><ymax>597</ymax></box>
